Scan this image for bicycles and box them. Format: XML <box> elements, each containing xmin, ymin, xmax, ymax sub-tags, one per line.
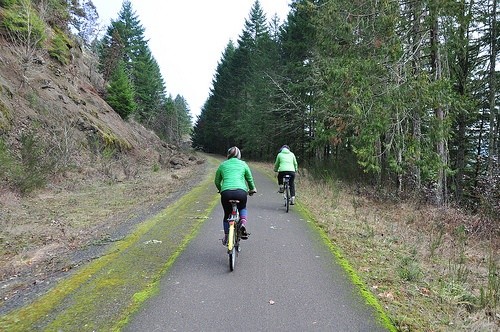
<box><xmin>216</xmin><ymin>189</ymin><xmax>257</xmax><ymax>272</ymax></box>
<box><xmin>278</xmin><ymin>168</ymin><xmax>295</xmax><ymax>214</ymax></box>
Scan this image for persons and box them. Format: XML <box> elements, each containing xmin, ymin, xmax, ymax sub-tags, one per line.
<box><xmin>215</xmin><ymin>147</ymin><xmax>257</xmax><ymax>245</ymax></box>
<box><xmin>273</xmin><ymin>145</ymin><xmax>298</xmax><ymax>205</ymax></box>
